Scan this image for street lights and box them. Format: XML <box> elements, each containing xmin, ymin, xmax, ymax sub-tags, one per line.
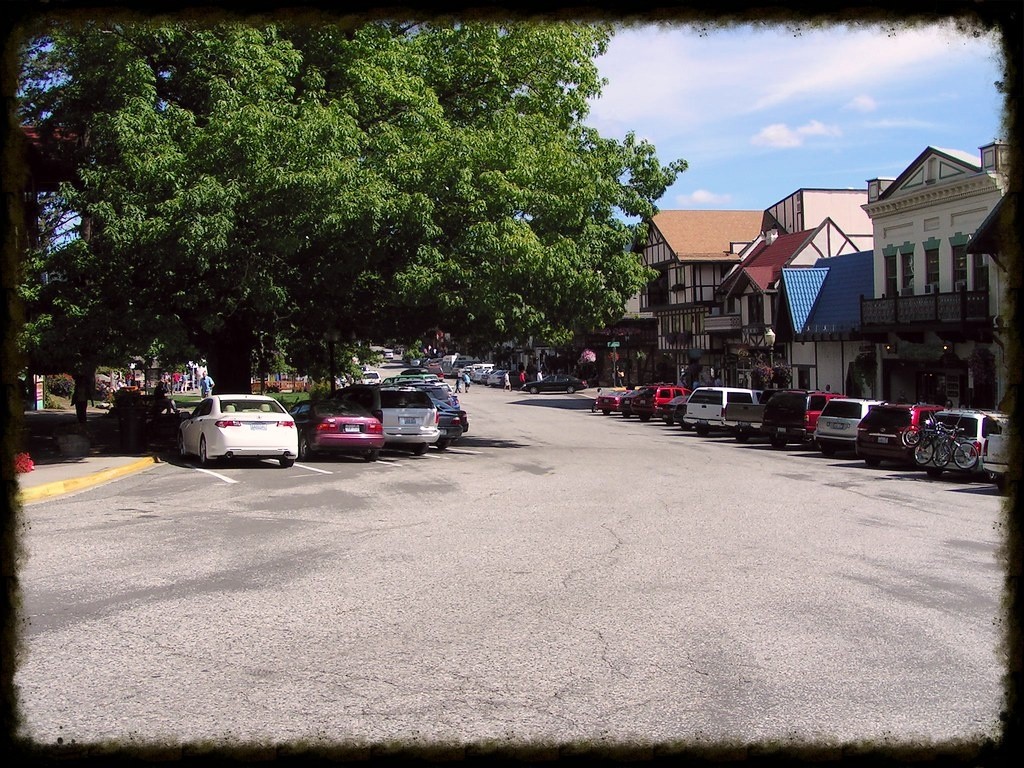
<box><xmin>764</xmin><ymin>327</ymin><xmax>775</xmax><ymax>369</ymax></box>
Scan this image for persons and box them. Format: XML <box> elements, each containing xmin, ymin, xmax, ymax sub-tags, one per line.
<box><xmin>692</xmin><ymin>376</ymin><xmax>721</xmax><ymax>390</ymax></box>
<box><xmin>765</xmin><ymin>377</ymin><xmax>773</xmax><ymax>389</ymax></box>
<box><xmin>200</xmin><ymin>371</ymin><xmax>215</xmax><ymax>400</ymax></box>
<box><xmin>99</xmin><ymin>380</ymin><xmax>108</xmax><ymax>392</ymax></box>
<box><xmin>536</xmin><ymin>371</ymin><xmax>542</xmax><ymax>381</ymax></box>
<box><xmin>916</xmin><ymin>395</ymin><xmax>927</xmax><ymax>406</ymax></box>
<box><xmin>117</xmin><ymin>379</ymin><xmax>122</xmax><ymax>387</ymax></box>
<box><xmin>504</xmin><ymin>371</ymin><xmax>512</xmax><ymax>392</ymax></box>
<box><xmin>153</xmin><ymin>381</ymin><xmax>181</xmax><ymax>415</ymax></box>
<box><xmin>125</xmin><ymin>371</ymin><xmax>130</xmax><ymax>386</ymax></box>
<box><xmin>464</xmin><ymin>373</ymin><xmax>470</xmax><ymax>393</ymax></box>
<box><xmin>742</xmin><ymin>375</ymin><xmax>749</xmax><ymax>389</ymax></box>
<box><xmin>615</xmin><ymin>365</ymin><xmax>624</xmax><ymax>387</ymax></box>
<box><xmin>518</xmin><ymin>370</ymin><xmax>526</xmax><ymax>390</ymax></box>
<box><xmin>677</xmin><ymin>377</ymin><xmax>687</xmax><ymax>387</ymax></box>
<box><xmin>162</xmin><ymin>369</ymin><xmax>201</xmax><ymax>394</ymax></box>
<box><xmin>945</xmin><ymin>400</ymin><xmax>954</xmax><ymax>409</ymax></box>
<box><xmin>454</xmin><ymin>378</ymin><xmax>461</xmax><ymax>393</ymax></box>
<box><xmin>825</xmin><ymin>384</ymin><xmax>830</xmax><ymax>393</ymax></box>
<box><xmin>132</xmin><ymin>371</ymin><xmax>136</xmax><ymax>386</ymax></box>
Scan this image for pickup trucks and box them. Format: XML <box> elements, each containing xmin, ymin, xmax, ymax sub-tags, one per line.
<box><xmin>980</xmin><ymin>432</ymin><xmax>1008</xmax><ymax>492</ymax></box>
<box><xmin>722</xmin><ymin>389</ymin><xmax>788</xmax><ymax>441</ymax></box>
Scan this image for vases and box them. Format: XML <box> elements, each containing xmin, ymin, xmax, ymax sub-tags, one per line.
<box><xmin>778</xmin><ymin>380</ymin><xmax>788</xmax><ymax>388</ymax></box>
<box><xmin>759</xmin><ymin>382</ymin><xmax>769</xmax><ymax>390</ymax></box>
<box><xmin>693</xmin><ymin>380</ymin><xmax>700</xmax><ymax>390</ymax></box>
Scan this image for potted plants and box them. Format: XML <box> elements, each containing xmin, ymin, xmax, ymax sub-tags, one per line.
<box><xmin>853</xmin><ymin>352</ymin><xmax>878</xmax><ymax>393</ymax></box>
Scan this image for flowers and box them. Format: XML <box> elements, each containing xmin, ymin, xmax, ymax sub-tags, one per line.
<box><xmin>689</xmin><ymin>364</ymin><xmax>703</xmax><ymax>381</ymax></box>
<box><xmin>968</xmin><ymin>348</ymin><xmax>996</xmax><ymax>384</ymax></box>
<box><xmin>754</xmin><ymin>363</ymin><xmax>774</xmax><ymax>381</ymax></box>
<box><xmin>773</xmin><ymin>363</ymin><xmax>791</xmax><ymax>384</ymax></box>
<box><xmin>665</xmin><ymin>331</ymin><xmax>691</xmax><ymax>346</ymax></box>
<box><xmin>672</xmin><ymin>283</ymin><xmax>683</xmax><ymax>292</ymax></box>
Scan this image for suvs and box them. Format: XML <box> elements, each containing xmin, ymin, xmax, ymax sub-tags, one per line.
<box><xmin>914</xmin><ymin>406</ymin><xmax>1009</xmax><ymax>480</ymax></box>
<box><xmin>760</xmin><ymin>388</ymin><xmax>849</xmax><ymax>449</ymax></box>
<box><xmin>815</xmin><ymin>398</ymin><xmax>891</xmax><ymax>460</ymax></box>
<box><xmin>683</xmin><ymin>386</ymin><xmax>764</xmax><ymax>437</ymax></box>
<box><xmin>629</xmin><ymin>381</ymin><xmax>693</xmax><ymax>420</ymax></box>
<box><xmin>853</xmin><ymin>399</ymin><xmax>961</xmax><ymax>466</ymax></box>
<box><xmin>329</xmin><ymin>383</ymin><xmax>442</xmax><ymax>454</ymax></box>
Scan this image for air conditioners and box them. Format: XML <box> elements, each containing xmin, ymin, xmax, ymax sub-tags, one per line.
<box><xmin>924</xmin><ymin>284</ymin><xmax>934</xmax><ymax>294</ymax></box>
<box><xmin>955</xmin><ymin>282</ymin><xmax>965</xmax><ymax>292</ymax></box>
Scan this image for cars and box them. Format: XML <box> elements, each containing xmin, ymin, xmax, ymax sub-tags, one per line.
<box><xmin>384</xmin><ymin>351</ymin><xmax>395</xmax><ymax>360</ymax></box>
<box><xmin>358</xmin><ymin>354</ymin><xmax>531</xmax><ymax>451</ymax></box>
<box><xmin>519</xmin><ymin>373</ymin><xmax>588</xmax><ymax>394</ymax></box>
<box><xmin>288</xmin><ymin>398</ymin><xmax>387</xmax><ymax>462</ymax></box>
<box><xmin>660</xmin><ymin>396</ymin><xmax>690</xmax><ymax>425</ymax></box>
<box><xmin>175</xmin><ymin>394</ymin><xmax>298</xmax><ymax>468</ymax></box>
<box><xmin>591</xmin><ymin>387</ymin><xmax>641</xmax><ymax>418</ymax></box>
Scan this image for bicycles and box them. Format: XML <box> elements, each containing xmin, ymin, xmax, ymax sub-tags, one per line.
<box><xmin>901</xmin><ymin>408</ymin><xmax>979</xmax><ymax>470</ymax></box>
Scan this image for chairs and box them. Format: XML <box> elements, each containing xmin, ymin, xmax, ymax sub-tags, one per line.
<box><xmin>260</xmin><ymin>404</ymin><xmax>270</xmax><ymax>412</ymax></box>
<box><xmin>225</xmin><ymin>405</ymin><xmax>235</xmax><ymax>412</ymax></box>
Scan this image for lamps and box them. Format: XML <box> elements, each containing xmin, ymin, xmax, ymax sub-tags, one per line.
<box><xmin>941</xmin><ymin>340</ymin><xmax>950</xmax><ymax>351</ymax></box>
<box><xmin>883</xmin><ymin>342</ymin><xmax>897</xmax><ymax>355</ymax></box>
